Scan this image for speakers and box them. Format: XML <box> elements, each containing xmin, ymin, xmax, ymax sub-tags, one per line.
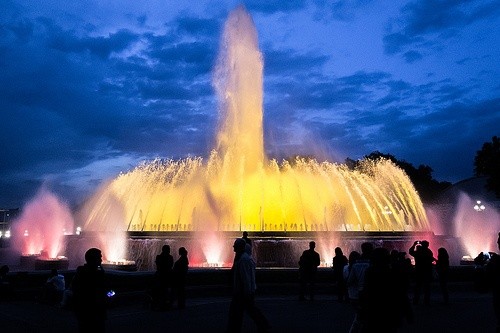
<box><xmin>460</xmin><ymin>255</ymin><xmax>474</xmax><ymax>265</ymax></box>
<box><xmin>36</xmin><ymin>258</ymin><xmax>69</xmax><ymax>271</ymax></box>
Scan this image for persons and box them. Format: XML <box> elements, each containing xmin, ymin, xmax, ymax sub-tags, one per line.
<box><xmin>0</xmin><ymin>232</ymin><xmax>500</xmax><ymax>333</ymax></box>
